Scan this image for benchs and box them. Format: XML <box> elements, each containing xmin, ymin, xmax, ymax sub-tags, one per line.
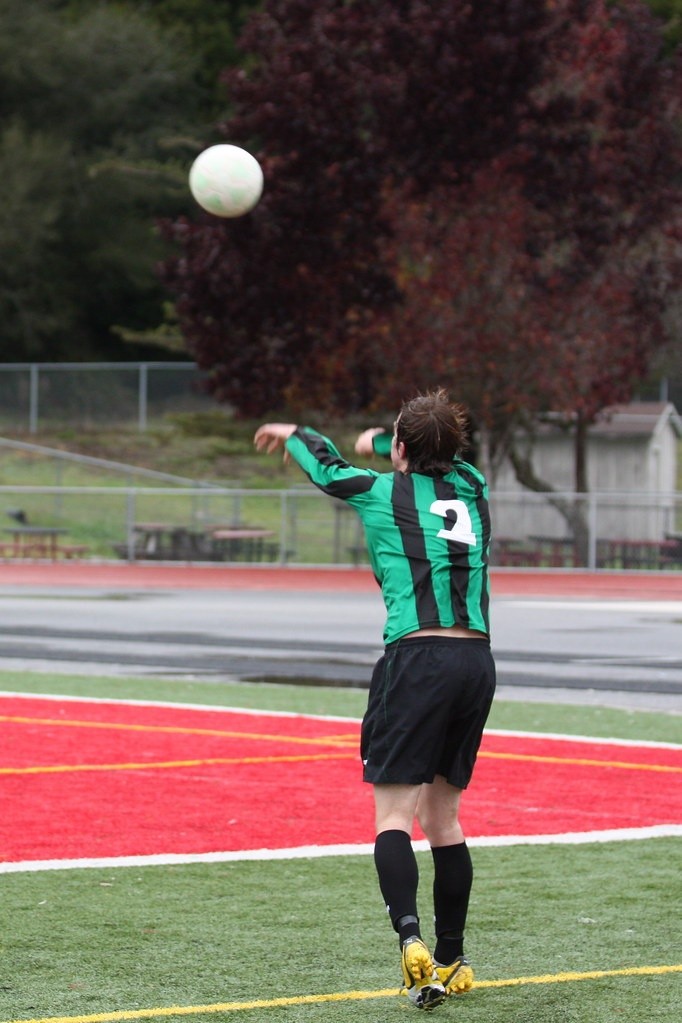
<box><xmin>114</xmin><ymin>541</ymin><xmax>298</xmax><ymax>563</ymax></box>
<box><xmin>491</xmin><ymin>550</ymin><xmax>679</xmax><ymax>569</ymax></box>
<box><xmin>0</xmin><ymin>544</ymin><xmax>89</xmax><ymax>559</ymax></box>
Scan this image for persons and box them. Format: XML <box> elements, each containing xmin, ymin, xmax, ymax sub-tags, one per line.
<box><xmin>253</xmin><ymin>393</ymin><xmax>496</xmax><ymax>1010</ymax></box>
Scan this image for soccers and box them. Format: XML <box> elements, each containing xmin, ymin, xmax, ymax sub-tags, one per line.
<box><xmin>188</xmin><ymin>143</ymin><xmax>266</xmax><ymax>220</ymax></box>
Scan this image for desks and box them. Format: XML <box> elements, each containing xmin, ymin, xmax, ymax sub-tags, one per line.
<box><xmin>610</xmin><ymin>540</ymin><xmax>679</xmax><ymax>569</ymax></box>
<box><xmin>4</xmin><ymin>527</ymin><xmax>71</xmax><ymax>558</ymax></box>
<box><xmin>136</xmin><ymin>522</ymin><xmax>279</xmax><ymax>563</ymax></box>
<box><xmin>593</xmin><ymin>539</ymin><xmax>610</xmax><ymax>567</ymax></box>
<box><xmin>528</xmin><ymin>534</ymin><xmax>578</xmax><ymax>565</ymax></box>
<box><xmin>491</xmin><ymin>538</ymin><xmax>523</xmax><ymax>567</ymax></box>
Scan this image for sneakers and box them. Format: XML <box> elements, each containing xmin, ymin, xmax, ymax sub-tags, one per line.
<box><xmin>400</xmin><ymin>958</ymin><xmax>473</xmax><ymax>997</ymax></box>
<box><xmin>398</xmin><ymin>936</ymin><xmax>446</xmax><ymax>1011</ymax></box>
